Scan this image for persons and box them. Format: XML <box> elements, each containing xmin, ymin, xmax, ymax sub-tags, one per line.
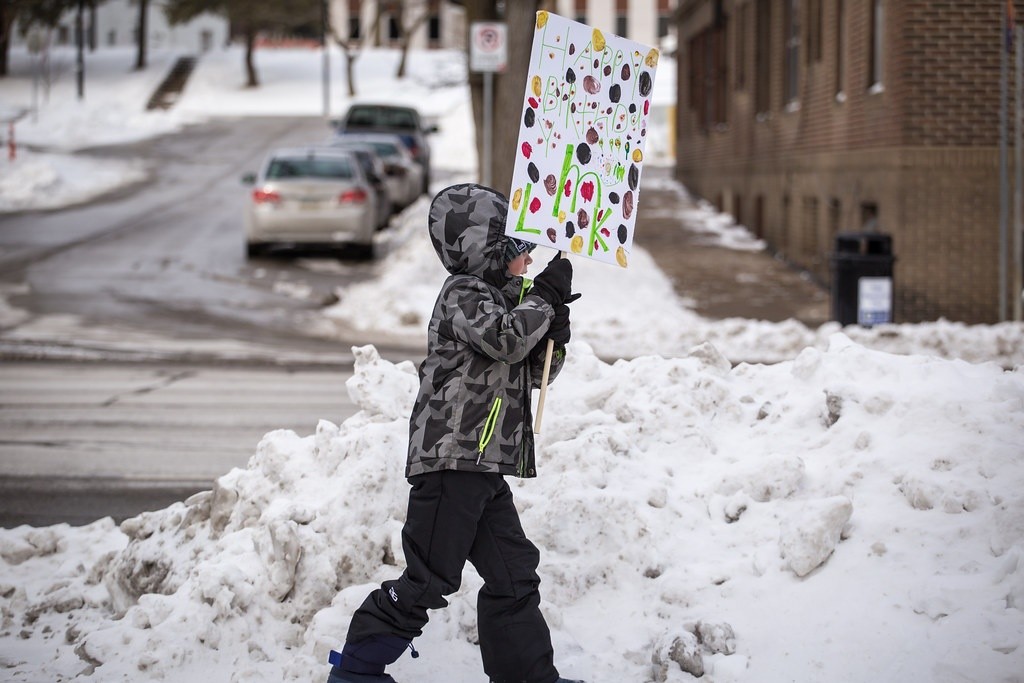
<box><xmin>326</xmin><ymin>186</ymin><xmax>582</xmax><ymax>683</ymax></box>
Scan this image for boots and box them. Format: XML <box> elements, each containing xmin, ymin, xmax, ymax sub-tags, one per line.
<box><xmin>326</xmin><ymin>625</ymin><xmax>420</xmax><ymax>683</ymax></box>
<box><xmin>490</xmin><ymin>675</ymin><xmax>584</xmax><ymax>683</ymax></box>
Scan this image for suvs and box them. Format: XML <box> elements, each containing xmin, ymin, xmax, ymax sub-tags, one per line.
<box><xmin>335</xmin><ymin>100</ymin><xmax>442</xmax><ymax>197</ymax></box>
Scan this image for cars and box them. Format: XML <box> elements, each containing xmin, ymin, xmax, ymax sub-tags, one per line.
<box><xmin>332</xmin><ymin>131</ymin><xmax>426</xmax><ymax>212</ymax></box>
<box><xmin>241</xmin><ymin>146</ymin><xmax>385</xmax><ymax>262</ymax></box>
<box><xmin>343</xmin><ymin>144</ymin><xmax>394</xmax><ymax>230</ymax></box>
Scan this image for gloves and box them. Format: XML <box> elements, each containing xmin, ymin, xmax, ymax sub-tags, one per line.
<box><xmin>531</xmin><ymin>251</ymin><xmax>579</xmax><ymax>345</ymax></box>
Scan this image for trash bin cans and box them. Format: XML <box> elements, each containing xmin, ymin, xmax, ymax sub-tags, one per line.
<box><xmin>827</xmin><ymin>232</ymin><xmax>896</xmax><ymax>327</ymax></box>
